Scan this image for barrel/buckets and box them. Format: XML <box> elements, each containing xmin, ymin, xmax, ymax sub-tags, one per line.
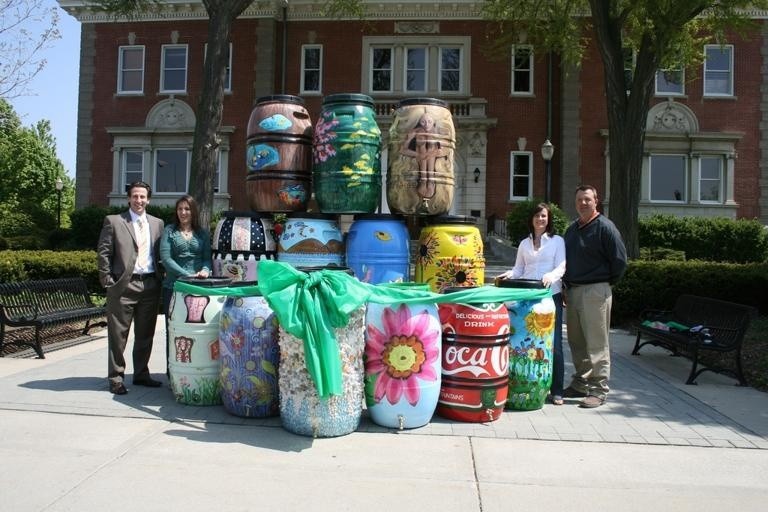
<box><xmin>364</xmin><ymin>302</ymin><xmax>443</xmax><ymax>431</ymax></box>
<box><xmin>276</xmin><ymin>302</ymin><xmax>366</xmax><ymax>437</ymax></box>
<box><xmin>311</xmin><ymin>93</ymin><xmax>384</xmax><ymax>215</ymax></box>
<box><xmin>242</xmin><ymin>94</ymin><xmax>314</xmax><ymax>215</ymax></box>
<box><xmin>438</xmin><ymin>300</ymin><xmax>511</xmax><ymax>425</ymax></box>
<box><xmin>385</xmin><ymin>97</ymin><xmax>456</xmax><ymax>216</ymax></box>
<box><xmin>168</xmin><ymin>277</ymin><xmax>235</xmax><ymax>409</ymax></box>
<box><xmin>506</xmin><ymin>296</ymin><xmax>557</xmax><ymax>412</ymax></box>
<box><xmin>344</xmin><ymin>214</ymin><xmax>412</xmax><ymax>285</ymax></box>
<box><xmin>217</xmin><ymin>278</ymin><xmax>280</xmax><ymax>420</ymax></box>
<box><xmin>277</xmin><ymin>210</ymin><xmax>343</xmax><ymax>272</ymax></box>
<box><xmin>415</xmin><ymin>215</ymin><xmax>486</xmax><ymax>294</ymax></box>
<box><xmin>211</xmin><ymin>210</ymin><xmax>278</xmax><ymax>281</ymax></box>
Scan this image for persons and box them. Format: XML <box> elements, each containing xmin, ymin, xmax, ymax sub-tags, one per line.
<box><xmin>96</xmin><ymin>182</ymin><xmax>165</xmax><ymax>394</ymax></box>
<box><xmin>398</xmin><ymin>115</ymin><xmax>451</xmax><ymax>199</ymax></box>
<box><xmin>493</xmin><ymin>201</ymin><xmax>566</xmax><ymax>408</ymax></box>
<box><xmin>562</xmin><ymin>183</ymin><xmax>629</xmax><ymax>408</ymax></box>
<box><xmin>158</xmin><ymin>194</ymin><xmax>211</xmax><ymax>380</ymax></box>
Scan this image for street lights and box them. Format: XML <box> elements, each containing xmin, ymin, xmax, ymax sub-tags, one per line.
<box><xmin>56</xmin><ymin>177</ymin><xmax>65</xmax><ymax>228</ymax></box>
<box><xmin>541</xmin><ymin>139</ymin><xmax>555</xmax><ymax>202</ymax></box>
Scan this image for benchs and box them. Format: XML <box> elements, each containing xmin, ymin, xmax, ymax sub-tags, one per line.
<box><xmin>626</xmin><ymin>287</ymin><xmax>761</xmax><ymax>391</ymax></box>
<box><xmin>0</xmin><ymin>266</ymin><xmax>118</xmax><ymax>370</ymax></box>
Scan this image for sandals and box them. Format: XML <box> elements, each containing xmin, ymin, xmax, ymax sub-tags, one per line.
<box><xmin>552</xmin><ymin>394</ymin><xmax>564</xmax><ymax>404</ymax></box>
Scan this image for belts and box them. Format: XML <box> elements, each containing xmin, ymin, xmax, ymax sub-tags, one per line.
<box><xmin>132</xmin><ymin>273</ymin><xmax>150</xmax><ymax>281</ymax></box>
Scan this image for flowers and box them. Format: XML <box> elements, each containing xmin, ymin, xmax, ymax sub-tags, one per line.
<box><xmin>365</xmin><ymin>301</ymin><xmax>443</xmax><ymax>409</ymax></box>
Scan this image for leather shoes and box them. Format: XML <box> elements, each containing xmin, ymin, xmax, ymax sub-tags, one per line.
<box><xmin>580</xmin><ymin>395</ymin><xmax>607</xmax><ymax>407</ymax></box>
<box><xmin>133</xmin><ymin>377</ymin><xmax>162</xmax><ymax>387</ymax></box>
<box><xmin>110</xmin><ymin>383</ymin><xmax>127</xmax><ymax>394</ymax></box>
<box><xmin>562</xmin><ymin>387</ymin><xmax>587</xmax><ymax>397</ymax></box>
